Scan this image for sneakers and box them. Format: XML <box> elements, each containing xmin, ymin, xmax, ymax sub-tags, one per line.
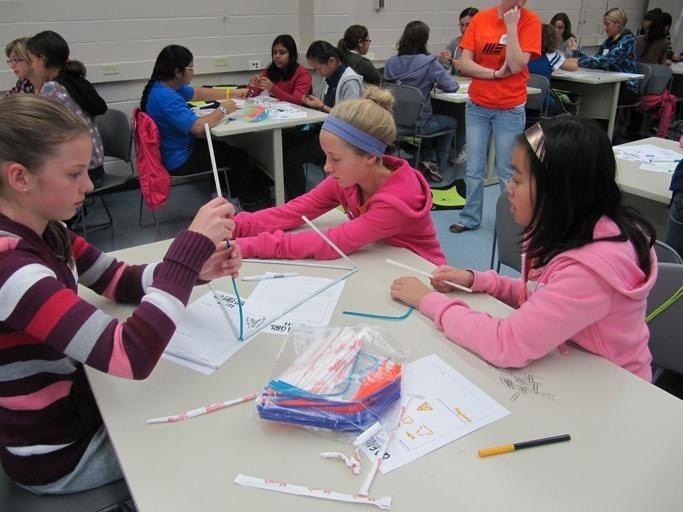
<box><xmin>451</xmin><ymin>145</ymin><xmax>466</xmax><ymax>165</ymax></box>
<box><xmin>420</xmin><ymin>159</ymin><xmax>444</xmax><ymax>184</ymax></box>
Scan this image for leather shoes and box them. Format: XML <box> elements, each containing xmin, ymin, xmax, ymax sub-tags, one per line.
<box><xmin>449</xmin><ymin>223</ymin><xmax>467</xmax><ymax>232</ymax></box>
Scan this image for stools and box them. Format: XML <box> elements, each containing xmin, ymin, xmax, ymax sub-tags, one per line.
<box><xmin>0</xmin><ymin>462</ymin><xmax>130</xmax><ymax>510</ymax></box>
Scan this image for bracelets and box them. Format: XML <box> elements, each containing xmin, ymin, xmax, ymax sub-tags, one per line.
<box><xmin>226</xmin><ymin>87</ymin><xmax>229</xmax><ymax>100</ymax></box>
<box><xmin>217</xmin><ymin>104</ymin><xmax>227</xmax><ymax>117</ymax></box>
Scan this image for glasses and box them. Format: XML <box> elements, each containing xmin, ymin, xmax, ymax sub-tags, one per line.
<box><xmin>181</xmin><ymin>66</ymin><xmax>195</xmax><ymax>75</ymax></box>
<box><xmin>457</xmin><ymin>23</ymin><xmax>470</xmax><ymax>28</ymax></box>
<box><xmin>554</xmin><ymin>25</ymin><xmax>566</xmax><ymax>29</ymax></box>
<box><xmin>5</xmin><ymin>58</ymin><xmax>28</xmax><ymax>65</ymax></box>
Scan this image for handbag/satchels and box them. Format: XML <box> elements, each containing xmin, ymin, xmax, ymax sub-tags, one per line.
<box><xmin>549</xmin><ymin>88</ymin><xmax>583</xmax><ymax>115</ymax></box>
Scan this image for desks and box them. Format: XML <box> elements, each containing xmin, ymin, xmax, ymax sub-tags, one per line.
<box><xmin>551</xmin><ymin>66</ymin><xmax>646</xmax><ymax>145</ymax></box>
<box><xmin>661</xmin><ymin>62</ymin><xmax>683</xmax><ymax>75</ymax></box>
<box><xmin>429</xmin><ymin>74</ymin><xmax>542</xmax><ymax>187</ymax></box>
<box><xmin>609</xmin><ymin>135</ymin><xmax>683</xmax><ymax>236</ymax></box>
<box><xmin>189</xmin><ymin>95</ymin><xmax>330</xmax><ymax>206</ymax></box>
<box><xmin>77</xmin><ymin>207</ymin><xmax>683</xmax><ymax>511</ymax></box>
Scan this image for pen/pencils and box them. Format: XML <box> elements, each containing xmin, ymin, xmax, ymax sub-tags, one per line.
<box><xmin>477</xmin><ymin>434</ymin><xmax>571</xmax><ymax>457</ymax></box>
<box><xmin>242</xmin><ymin>273</ymin><xmax>301</xmax><ymax>281</ymax></box>
<box><xmin>226</xmin><ymin>89</ymin><xmax>229</xmax><ymax>101</ymax></box>
<box><xmin>650</xmin><ymin>159</ymin><xmax>681</xmax><ymax>162</ymax></box>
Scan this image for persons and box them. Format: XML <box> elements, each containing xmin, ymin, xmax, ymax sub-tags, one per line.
<box><xmin>0</xmin><ymin>92</ymin><xmax>242</xmax><ymax>497</ymax></box>
<box><xmin>440</xmin><ymin>9</ymin><xmax>479</xmax><ymax>73</ymax></box>
<box><xmin>233</xmin><ymin>84</ymin><xmax>447</xmax><ymax>267</ymax></box>
<box><xmin>27</xmin><ymin>29</ymin><xmax>108</xmax><ymax>229</ymax></box>
<box><xmin>5</xmin><ymin>37</ymin><xmax>42</xmax><ymax>95</ymax></box>
<box><xmin>449</xmin><ymin>0</ymin><xmax>543</xmax><ymax>234</ymax></box>
<box><xmin>390</xmin><ymin>117</ymin><xmax>658</xmax><ymax>383</ymax></box>
<box><xmin>140</xmin><ymin>44</ymin><xmax>270</xmax><ymax>205</ymax></box>
<box><xmin>384</xmin><ymin>20</ymin><xmax>459</xmax><ymax>179</ymax></box>
<box><xmin>530</xmin><ymin>8</ymin><xmax>683</xmax><ymax>140</ymax></box>
<box><xmin>249</xmin><ymin>25</ymin><xmax>377</xmax><ymax>180</ymax></box>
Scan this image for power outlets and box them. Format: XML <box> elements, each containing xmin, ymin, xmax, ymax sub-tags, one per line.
<box><xmin>248</xmin><ymin>60</ymin><xmax>261</xmax><ymax>71</ymax></box>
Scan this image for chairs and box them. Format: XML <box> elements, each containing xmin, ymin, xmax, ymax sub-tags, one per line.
<box><xmin>647</xmin><ymin>64</ymin><xmax>674</xmax><ymax>136</ymax></box>
<box><xmin>527</xmin><ymin>73</ymin><xmax>573</xmax><ymax>121</ymax></box>
<box><xmin>645</xmin><ymin>263</ymin><xmax>683</xmax><ymax>377</ymax></box>
<box><xmin>65</xmin><ymin>109</ymin><xmax>131</xmax><ymax>232</ymax></box>
<box><xmin>379</xmin><ymin>80</ymin><xmax>459</xmax><ymax>181</ymax></box>
<box><xmin>612</xmin><ymin>62</ymin><xmax>652</xmax><ymax>143</ymax></box>
<box><xmin>131</xmin><ymin>108</ymin><xmax>236</xmax><ymax>228</ymax></box>
<box><xmin>493</xmin><ymin>190</ymin><xmax>525</xmax><ymax>274</ymax></box>
<box><xmin>642</xmin><ymin>233</ymin><xmax>683</xmax><ymax>264</ymax></box>
